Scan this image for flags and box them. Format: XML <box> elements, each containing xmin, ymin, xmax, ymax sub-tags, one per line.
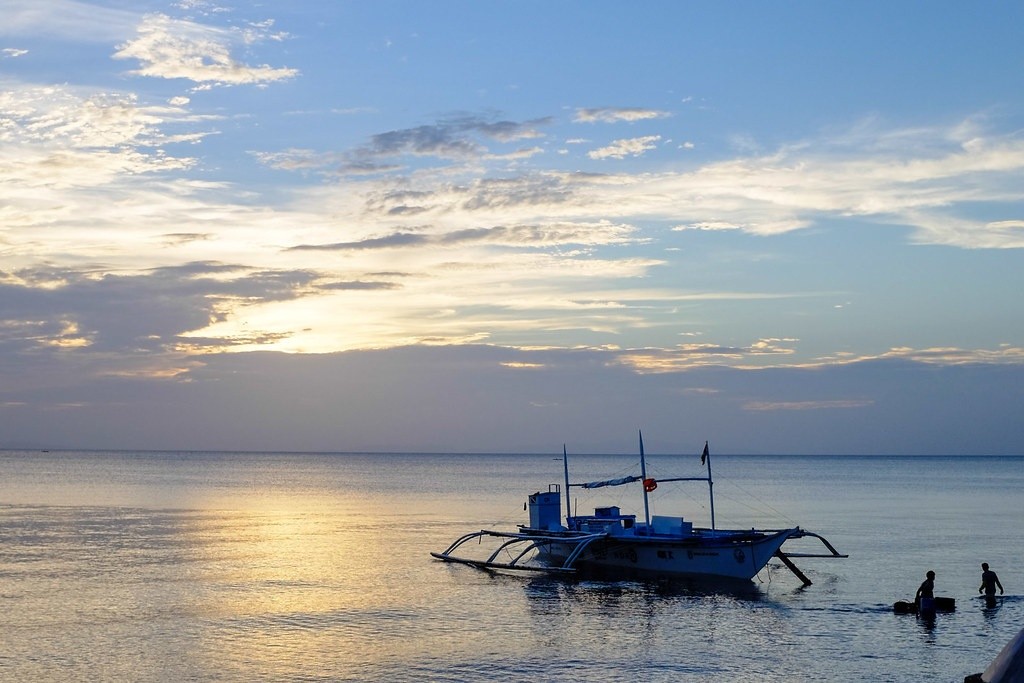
<box><xmin>700</xmin><ymin>443</ymin><xmax>709</xmax><ymax>465</ymax></box>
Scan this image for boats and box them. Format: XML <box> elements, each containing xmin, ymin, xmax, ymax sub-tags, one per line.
<box><xmin>430</xmin><ymin>428</ymin><xmax>849</xmax><ymax>587</ymax></box>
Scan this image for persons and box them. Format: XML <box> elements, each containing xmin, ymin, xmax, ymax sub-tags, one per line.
<box><xmin>915</xmin><ymin>571</ymin><xmax>936</xmax><ymax>621</ymax></box>
<box><xmin>979</xmin><ymin>563</ymin><xmax>1004</xmax><ymax>606</ymax></box>
